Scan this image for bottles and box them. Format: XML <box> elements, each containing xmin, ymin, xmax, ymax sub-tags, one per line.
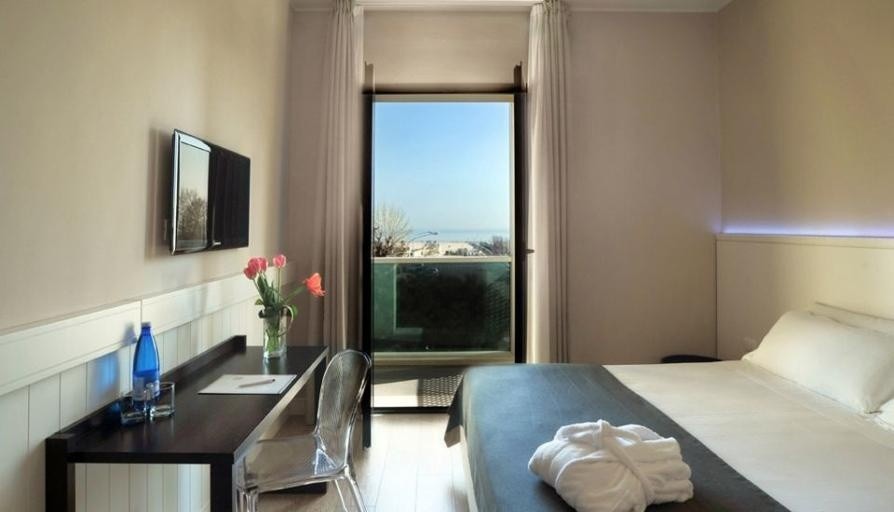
<box><xmin>133</xmin><ymin>322</ymin><xmax>160</xmax><ymax>409</ymax></box>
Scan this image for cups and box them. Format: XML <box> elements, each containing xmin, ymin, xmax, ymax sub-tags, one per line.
<box><xmin>119</xmin><ymin>389</ymin><xmax>147</xmax><ymax>423</ymax></box>
<box><xmin>147</xmin><ymin>381</ymin><xmax>175</xmax><ymax>418</ymax></box>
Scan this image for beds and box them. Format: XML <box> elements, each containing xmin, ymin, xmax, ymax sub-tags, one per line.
<box><xmin>443</xmin><ymin>301</ymin><xmax>894</xmax><ymax>512</ymax></box>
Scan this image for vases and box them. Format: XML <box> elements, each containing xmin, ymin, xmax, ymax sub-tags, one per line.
<box><xmin>262</xmin><ymin>308</ymin><xmax>287</xmax><ymax>359</ymax></box>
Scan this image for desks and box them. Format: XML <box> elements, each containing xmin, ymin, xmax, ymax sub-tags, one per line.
<box><xmin>45</xmin><ymin>335</ymin><xmax>329</xmax><ymax>512</ymax></box>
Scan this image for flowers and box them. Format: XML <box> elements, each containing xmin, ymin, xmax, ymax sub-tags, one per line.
<box><xmin>243</xmin><ymin>255</ymin><xmax>327</xmax><ymax>350</ymax></box>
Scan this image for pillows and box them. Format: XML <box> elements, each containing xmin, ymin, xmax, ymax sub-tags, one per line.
<box><xmin>741</xmin><ymin>306</ymin><xmax>894</xmax><ymax>431</ymax></box>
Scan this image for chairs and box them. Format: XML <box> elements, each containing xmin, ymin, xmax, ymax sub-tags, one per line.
<box><xmin>232</xmin><ymin>348</ymin><xmax>372</xmax><ymax>511</ymax></box>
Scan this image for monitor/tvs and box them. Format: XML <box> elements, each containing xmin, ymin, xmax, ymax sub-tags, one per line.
<box><xmin>169</xmin><ymin>128</ymin><xmax>251</xmax><ymax>257</ymax></box>
